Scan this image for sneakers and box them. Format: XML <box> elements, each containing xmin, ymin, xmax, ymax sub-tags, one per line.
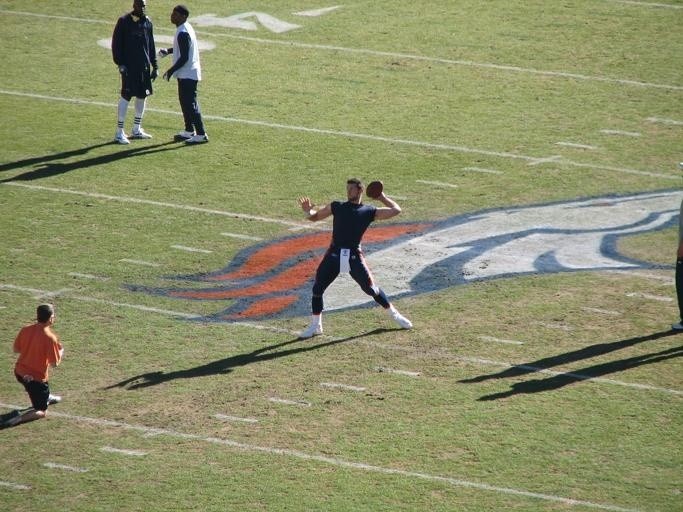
<box><xmin>47</xmin><ymin>394</ymin><xmax>61</xmax><ymax>404</ymax></box>
<box><xmin>391</xmin><ymin>311</ymin><xmax>413</xmax><ymax>329</ymax></box>
<box><xmin>115</xmin><ymin>132</ymin><xmax>130</xmax><ymax>144</ymax></box>
<box><xmin>0</xmin><ymin>410</ymin><xmax>22</xmax><ymax>429</ymax></box>
<box><xmin>185</xmin><ymin>133</ymin><xmax>209</xmax><ymax>144</ymax></box>
<box><xmin>174</xmin><ymin>130</ymin><xmax>195</xmax><ymax>142</ymax></box>
<box><xmin>672</xmin><ymin>324</ymin><xmax>683</xmax><ymax>330</ymax></box>
<box><xmin>300</xmin><ymin>325</ymin><xmax>323</xmax><ymax>337</ymax></box>
<box><xmin>132</xmin><ymin>128</ymin><xmax>152</xmax><ymax>139</ymax></box>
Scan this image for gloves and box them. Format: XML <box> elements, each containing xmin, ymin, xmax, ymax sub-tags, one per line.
<box><xmin>119</xmin><ymin>65</ymin><xmax>127</xmax><ymax>74</ymax></box>
<box><xmin>150</xmin><ymin>69</ymin><xmax>158</xmax><ymax>82</ymax></box>
<box><xmin>163</xmin><ymin>70</ymin><xmax>173</xmax><ymax>81</ymax></box>
<box><xmin>159</xmin><ymin>48</ymin><xmax>168</xmax><ymax>57</ymax></box>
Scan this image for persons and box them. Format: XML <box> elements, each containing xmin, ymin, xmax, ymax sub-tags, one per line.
<box><xmin>670</xmin><ymin>197</ymin><xmax>682</xmax><ymax>330</ymax></box>
<box><xmin>154</xmin><ymin>3</ymin><xmax>209</xmax><ymax>144</ymax></box>
<box><xmin>111</xmin><ymin>0</ymin><xmax>159</xmax><ymax>144</ymax></box>
<box><xmin>0</xmin><ymin>303</ymin><xmax>65</xmax><ymax>430</ymax></box>
<box><xmin>297</xmin><ymin>177</ymin><xmax>413</xmax><ymax>340</ymax></box>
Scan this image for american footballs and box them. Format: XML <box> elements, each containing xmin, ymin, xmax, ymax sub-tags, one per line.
<box><xmin>366</xmin><ymin>181</ymin><xmax>383</xmax><ymax>197</ymax></box>
